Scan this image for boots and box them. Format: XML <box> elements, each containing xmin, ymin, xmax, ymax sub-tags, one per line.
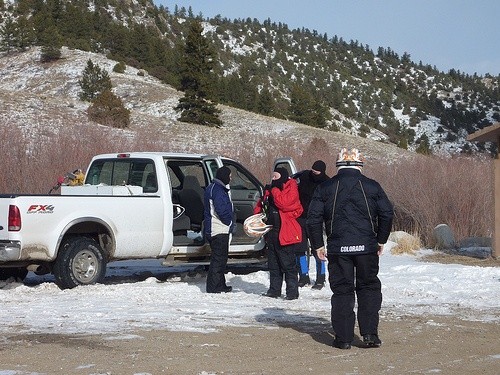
<box><xmin>298</xmin><ymin>274</ymin><xmax>310</xmax><ymax>287</ymax></box>
<box><xmin>312</xmin><ymin>274</ymin><xmax>325</xmax><ymax>290</ymax></box>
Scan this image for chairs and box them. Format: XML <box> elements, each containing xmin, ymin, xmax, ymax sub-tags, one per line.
<box><xmin>143</xmin><ymin>173</ymin><xmax>158</xmax><ymax>193</ymax></box>
<box><xmin>179</xmin><ymin>175</ymin><xmax>204</xmax><ymax>224</ymax></box>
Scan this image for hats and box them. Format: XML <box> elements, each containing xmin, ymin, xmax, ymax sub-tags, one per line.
<box><xmin>312</xmin><ymin>160</ymin><xmax>326</xmax><ymax>173</ymax></box>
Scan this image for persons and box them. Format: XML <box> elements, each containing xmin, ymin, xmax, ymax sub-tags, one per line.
<box><xmin>291</xmin><ymin>160</ymin><xmax>330</xmax><ymax>291</ymax></box>
<box><xmin>254</xmin><ymin>167</ymin><xmax>303</xmax><ymax>300</ymax></box>
<box><xmin>204</xmin><ymin>167</ymin><xmax>233</xmax><ymax>293</ymax></box>
<box><xmin>307</xmin><ymin>148</ymin><xmax>393</xmax><ymax>349</ymax></box>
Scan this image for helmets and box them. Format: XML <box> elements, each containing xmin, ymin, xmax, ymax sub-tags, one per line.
<box><xmin>243</xmin><ymin>213</ymin><xmax>273</xmax><ymax>238</ymax></box>
<box><xmin>336</xmin><ymin>148</ymin><xmax>364</xmax><ymax>172</ymax></box>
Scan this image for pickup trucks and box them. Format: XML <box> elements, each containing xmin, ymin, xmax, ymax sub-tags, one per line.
<box><xmin>0</xmin><ymin>151</ymin><xmax>297</xmax><ymax>290</ymax></box>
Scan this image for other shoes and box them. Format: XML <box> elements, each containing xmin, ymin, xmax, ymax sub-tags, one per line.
<box><xmin>364</xmin><ymin>335</ymin><xmax>381</xmax><ymax>346</ymax></box>
<box><xmin>332</xmin><ymin>339</ymin><xmax>351</xmax><ymax>349</ymax></box>
<box><xmin>262</xmin><ymin>291</ymin><xmax>281</xmax><ymax>298</ymax></box>
<box><xmin>207</xmin><ymin>286</ymin><xmax>232</xmax><ymax>293</ymax></box>
<box><xmin>284</xmin><ymin>296</ymin><xmax>299</xmax><ymax>300</ymax></box>
